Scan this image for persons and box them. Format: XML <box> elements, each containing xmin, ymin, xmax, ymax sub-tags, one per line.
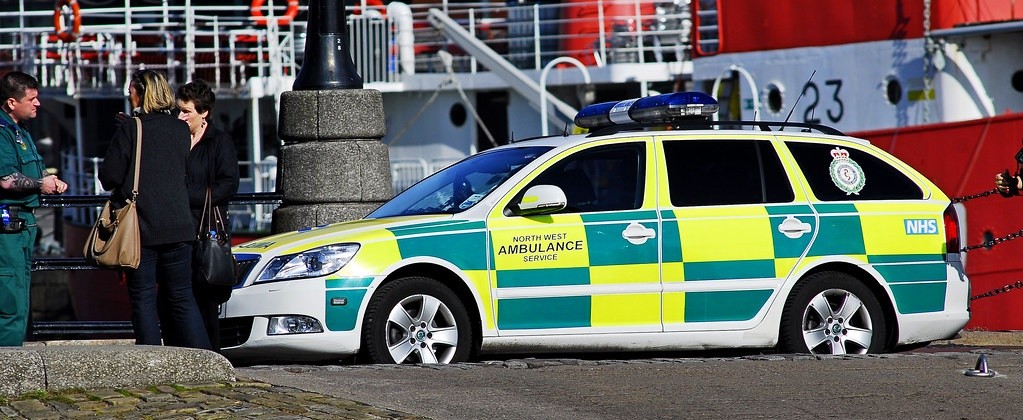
<box><xmin>0</xmin><ymin>71</ymin><xmax>68</xmax><ymax>347</ymax></box>
<box><xmin>97</xmin><ymin>68</ymin><xmax>241</xmax><ymax>353</ymax></box>
<box><xmin>995</xmin><ymin>148</ymin><xmax>1023</xmax><ymax>198</ymax></box>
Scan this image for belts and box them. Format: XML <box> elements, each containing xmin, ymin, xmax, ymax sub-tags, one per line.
<box><xmin>6</xmin><ymin>206</ymin><xmax>33</xmax><ymax>212</ymax></box>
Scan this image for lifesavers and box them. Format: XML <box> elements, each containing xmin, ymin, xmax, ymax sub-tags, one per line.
<box><xmin>353</xmin><ymin>1</ymin><xmax>387</xmax><ymax>16</ymax></box>
<box><xmin>53</xmin><ymin>1</ymin><xmax>82</xmax><ymax>42</ymax></box>
<box><xmin>250</xmin><ymin>0</ymin><xmax>299</xmax><ymax>27</ymax></box>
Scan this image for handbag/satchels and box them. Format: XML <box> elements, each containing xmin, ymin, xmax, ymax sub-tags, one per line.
<box><xmin>84</xmin><ymin>200</ymin><xmax>141</xmax><ymax>270</ymax></box>
<box><xmin>193</xmin><ymin>188</ymin><xmax>237</xmax><ymax>296</ymax></box>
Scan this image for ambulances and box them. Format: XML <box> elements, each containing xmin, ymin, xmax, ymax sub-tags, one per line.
<box><xmin>215</xmin><ymin>89</ymin><xmax>973</xmax><ymax>369</ymax></box>
